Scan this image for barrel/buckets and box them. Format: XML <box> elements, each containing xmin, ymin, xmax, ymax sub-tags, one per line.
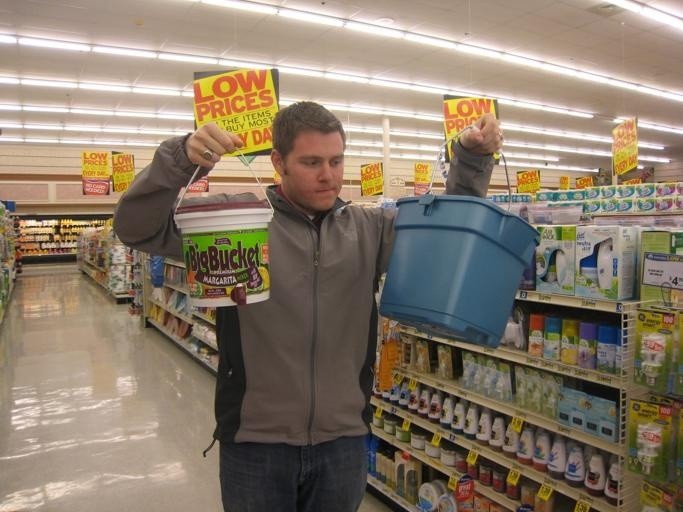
<box><xmin>379</xmin><ymin>127</ymin><xmax>542</xmax><ymax>348</ymax></box>
<box><xmin>173</xmin><ymin>148</ymin><xmax>274</xmax><ymax>310</ymax></box>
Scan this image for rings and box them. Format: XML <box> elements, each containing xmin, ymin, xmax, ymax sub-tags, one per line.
<box><xmin>499</xmin><ymin>133</ymin><xmax>504</xmax><ymax>140</ymax></box>
<box><xmin>113</xmin><ymin>110</ymin><xmax>504</xmax><ymax>511</ymax></box>
<box><xmin>201</xmin><ymin>151</ymin><xmax>213</xmax><ymax>163</ymax></box>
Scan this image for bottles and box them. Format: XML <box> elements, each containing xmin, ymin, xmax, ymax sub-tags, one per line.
<box><xmin>456</xmin><ymin>448</ymin><xmax>520</xmax><ymax>501</ymax></box>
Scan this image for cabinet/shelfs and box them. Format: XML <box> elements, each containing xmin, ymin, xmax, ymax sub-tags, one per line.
<box><xmin>123</xmin><ymin>207</ymin><xmax>683</xmax><ymax>508</ymax></box>
<box><xmin>1</xmin><ymin>207</ymin><xmax>25</xmax><ymax>326</ymax></box>
<box><xmin>75</xmin><ymin>218</ymin><xmax>132</xmax><ymax>306</ymax></box>
<box><xmin>14</xmin><ymin>212</ymin><xmax>114</xmax><ymax>266</ymax></box>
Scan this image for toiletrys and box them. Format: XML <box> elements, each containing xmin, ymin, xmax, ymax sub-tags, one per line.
<box><xmin>365</xmin><ymin>316</ymin><xmax>629</xmax><ymax>512</ymax></box>
